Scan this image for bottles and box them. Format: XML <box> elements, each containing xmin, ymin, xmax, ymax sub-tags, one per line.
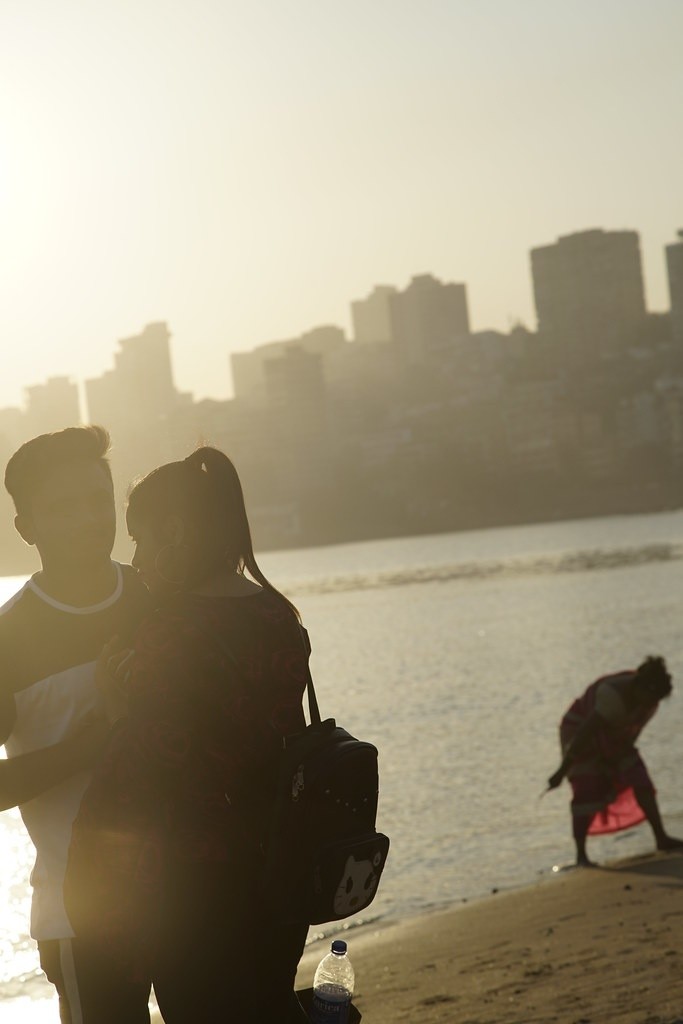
<box><xmin>311</xmin><ymin>940</ymin><xmax>355</xmax><ymax>1024</ymax></box>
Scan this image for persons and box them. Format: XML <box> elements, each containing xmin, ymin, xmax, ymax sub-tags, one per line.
<box><xmin>1</xmin><ymin>427</ymin><xmax>153</xmax><ymax>994</ymax></box>
<box><xmin>548</xmin><ymin>655</ymin><xmax>683</xmax><ymax>867</ymax></box>
<box><xmin>59</xmin><ymin>445</ymin><xmax>368</xmax><ymax>1024</ymax></box>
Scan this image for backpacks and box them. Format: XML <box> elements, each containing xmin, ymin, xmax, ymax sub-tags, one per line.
<box><xmin>251</xmin><ymin>666</ymin><xmax>390</xmax><ymax>925</ymax></box>
